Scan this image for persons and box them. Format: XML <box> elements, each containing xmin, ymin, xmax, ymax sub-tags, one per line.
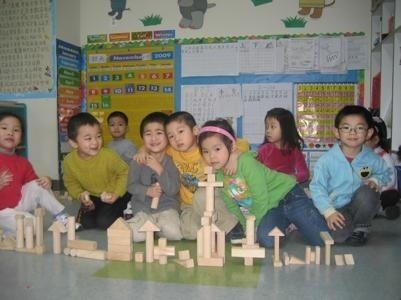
<box><xmin>309</xmin><ymin>105</ymin><xmax>391</xmax><ymax>246</ymax></box>
<box><xmin>63</xmin><ymin>112</ymin><xmax>129</xmax><ymax>230</ymax></box>
<box><xmin>0</xmin><ymin>112</ymin><xmax>84</xmax><ymax>239</ymax></box>
<box><xmin>106</xmin><ymin>111</ymin><xmax>138</xmax><ymax>166</ymax></box>
<box><xmin>255</xmin><ymin>108</ymin><xmax>309</xmax><ymax>189</ymax></box>
<box><xmin>364</xmin><ymin>117</ymin><xmax>400</xmax><ymax>219</ymax></box>
<box><xmin>133</xmin><ymin>111</ymin><xmax>250</xmax><ymax>242</ymax></box>
<box><xmin>197</xmin><ymin>119</ymin><xmax>332</xmax><ymax>249</ymax></box>
<box><xmin>126</xmin><ymin>111</ymin><xmax>182</xmax><ymax>244</ymax></box>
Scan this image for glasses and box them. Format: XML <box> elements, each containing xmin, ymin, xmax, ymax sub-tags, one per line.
<box><xmin>338</xmin><ymin>125</ymin><xmax>366</xmax><ymax>134</ymax></box>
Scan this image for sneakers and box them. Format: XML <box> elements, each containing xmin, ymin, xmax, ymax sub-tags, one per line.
<box><xmin>344</xmin><ymin>230</ymin><xmax>367</xmax><ymax>246</ymax></box>
<box><xmin>380</xmin><ymin>200</ymin><xmax>400</xmax><ymax>219</ymax></box>
<box><xmin>229</xmin><ymin>222</ymin><xmax>246</xmax><ymax>244</ymax></box>
<box><xmin>53</xmin><ymin>211</ymin><xmax>84</xmax><ymax>231</ymax></box>
<box><xmin>288</xmin><ymin>223</ymin><xmax>298</xmax><ymax>233</ymax></box>
